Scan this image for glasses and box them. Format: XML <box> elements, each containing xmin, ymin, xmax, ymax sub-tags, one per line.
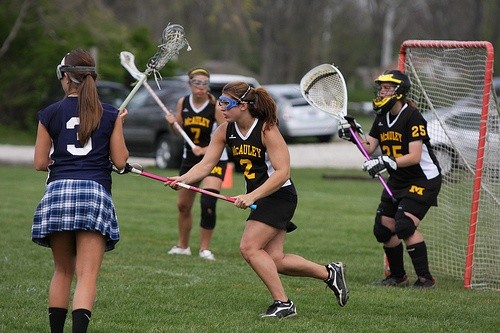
<box><xmin>190</xmin><ymin>79</ymin><xmax>210</xmax><ymax>89</ymax></box>
<box><xmin>219</xmin><ymin>96</ymin><xmax>241</xmax><ymax>110</ymax></box>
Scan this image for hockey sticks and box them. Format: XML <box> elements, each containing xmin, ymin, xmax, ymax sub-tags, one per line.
<box><xmin>117</xmin><ymin>24</ymin><xmax>187</xmax><ymax>113</ymax></box>
<box><xmin>119</xmin><ymin>52</ymin><xmax>196</xmax><ymax>150</ymax></box>
<box><xmin>109</xmin><ymin>159</ymin><xmax>257</xmax><ymax>210</ymax></box>
<box><xmin>300</xmin><ymin>63</ymin><xmax>397</xmax><ymax>203</ymax></box>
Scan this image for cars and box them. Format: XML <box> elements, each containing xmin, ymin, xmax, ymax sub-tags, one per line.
<box><xmin>261</xmin><ymin>83</ymin><xmax>338</xmax><ymax>141</ymax></box>
<box><xmin>420</xmin><ymin>96</ymin><xmax>498</xmax><ymax>178</ymax></box>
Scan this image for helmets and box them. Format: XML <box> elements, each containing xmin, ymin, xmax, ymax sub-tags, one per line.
<box><xmin>372</xmin><ymin>70</ymin><xmax>411</xmax><ymax>117</ymax></box>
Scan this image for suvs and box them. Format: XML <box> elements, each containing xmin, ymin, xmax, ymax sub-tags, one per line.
<box><xmin>117</xmin><ymin>73</ymin><xmax>261</xmax><ymax>170</ymax></box>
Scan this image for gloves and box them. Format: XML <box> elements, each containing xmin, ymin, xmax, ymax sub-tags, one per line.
<box><xmin>338</xmin><ymin>116</ymin><xmax>367</xmax><ymax>145</ymax></box>
<box><xmin>363</xmin><ymin>155</ymin><xmax>398</xmax><ymax>178</ymax></box>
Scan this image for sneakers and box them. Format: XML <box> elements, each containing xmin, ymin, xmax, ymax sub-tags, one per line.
<box><xmin>373</xmin><ymin>275</ymin><xmax>409</xmax><ymax>287</ymax></box>
<box><xmin>324</xmin><ymin>261</ymin><xmax>350</xmax><ymax>307</ymax></box>
<box><xmin>258</xmin><ymin>301</ymin><xmax>297</xmax><ymax>318</ymax></box>
<box><xmin>411</xmin><ymin>277</ymin><xmax>437</xmax><ymax>289</ymax></box>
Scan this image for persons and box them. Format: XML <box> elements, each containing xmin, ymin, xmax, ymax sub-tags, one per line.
<box><xmin>164</xmin><ymin>81</ymin><xmax>349</xmax><ymax>319</ymax></box>
<box><xmin>32</xmin><ymin>48</ymin><xmax>129</xmax><ymax>333</ymax></box>
<box><xmin>164</xmin><ymin>67</ymin><xmax>231</xmax><ymax>260</ymax></box>
<box><xmin>338</xmin><ymin>70</ymin><xmax>442</xmax><ymax>288</ymax></box>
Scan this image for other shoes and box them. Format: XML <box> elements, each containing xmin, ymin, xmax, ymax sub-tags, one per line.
<box><xmin>167</xmin><ymin>246</ymin><xmax>191</xmax><ymax>255</ymax></box>
<box><xmin>199</xmin><ymin>249</ymin><xmax>215</xmax><ymax>261</ymax></box>
